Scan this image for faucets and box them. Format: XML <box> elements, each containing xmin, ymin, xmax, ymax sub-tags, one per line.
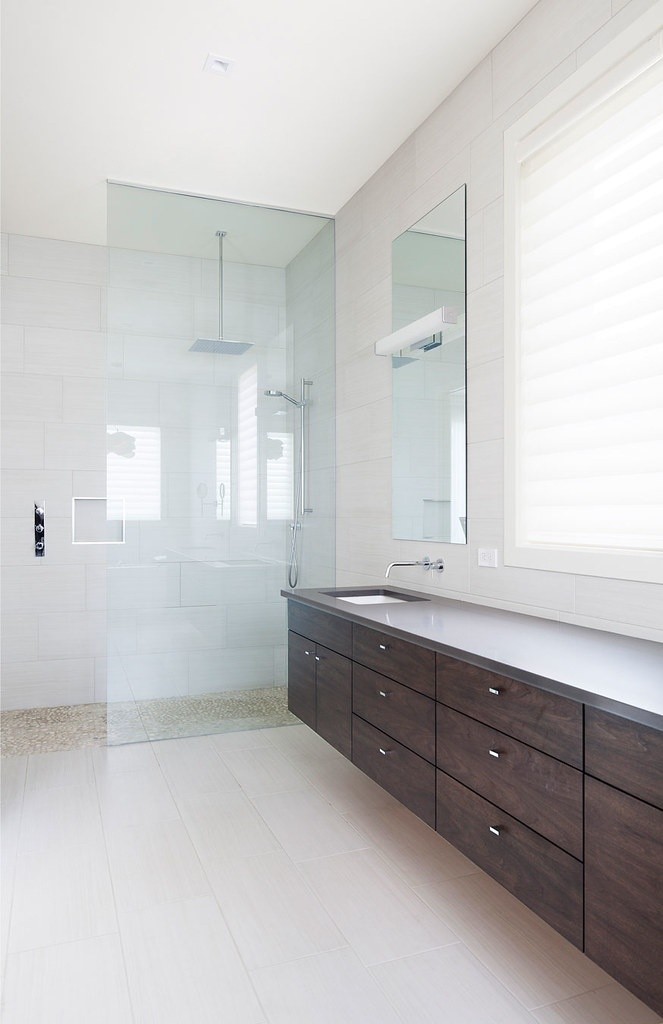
<box><xmin>384</xmin><ymin>560</ymin><xmax>430</xmax><ymax>578</ymax></box>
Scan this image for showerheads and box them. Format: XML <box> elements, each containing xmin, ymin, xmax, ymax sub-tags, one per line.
<box><xmin>187</xmin><ymin>336</ymin><xmax>255</xmax><ymax>357</ymax></box>
<box><xmin>263</xmin><ymin>388</ymin><xmax>300</xmax><ymax>406</ymax></box>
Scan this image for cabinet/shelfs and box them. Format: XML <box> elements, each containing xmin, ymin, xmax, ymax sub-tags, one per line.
<box><xmin>280</xmin><ymin>583</ymin><xmax>663</xmax><ymax>1018</ymax></box>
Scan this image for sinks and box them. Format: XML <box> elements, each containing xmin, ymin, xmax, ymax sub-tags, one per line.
<box><xmin>318</xmin><ymin>588</ymin><xmax>432</xmax><ymax>606</ymax></box>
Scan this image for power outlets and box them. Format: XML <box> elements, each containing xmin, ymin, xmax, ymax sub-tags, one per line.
<box><xmin>477</xmin><ymin>548</ymin><xmax>498</xmax><ymax>568</ymax></box>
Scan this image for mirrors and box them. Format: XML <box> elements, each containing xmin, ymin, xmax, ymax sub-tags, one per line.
<box><xmin>389</xmin><ymin>182</ymin><xmax>468</xmax><ymax>545</ymax></box>
